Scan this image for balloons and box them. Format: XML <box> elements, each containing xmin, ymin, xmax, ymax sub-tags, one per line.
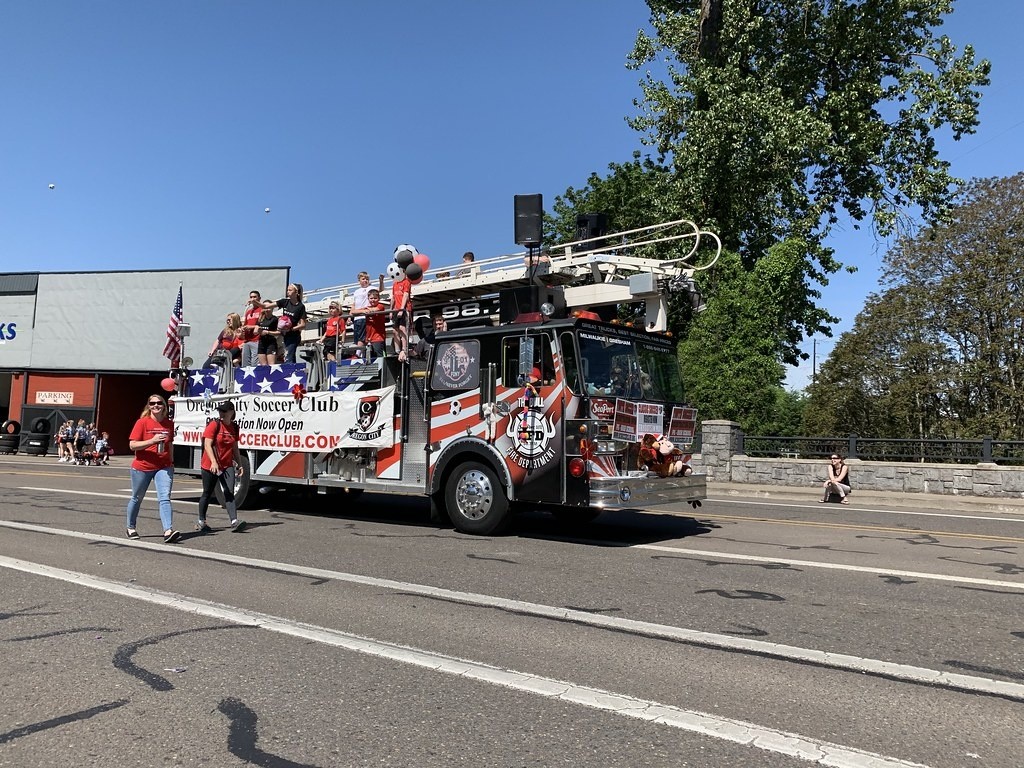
<box><xmin>387</xmin><ymin>245</ymin><xmax>430</xmax><ymax>285</ymax></box>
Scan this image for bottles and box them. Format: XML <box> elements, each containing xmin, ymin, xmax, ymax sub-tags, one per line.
<box><xmin>157</xmin><ymin>441</ymin><xmax>165</xmax><ymax>453</ymax></box>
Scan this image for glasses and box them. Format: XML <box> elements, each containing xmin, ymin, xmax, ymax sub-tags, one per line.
<box><xmin>218</xmin><ymin>410</ymin><xmax>227</xmax><ymax>413</ymax></box>
<box><xmin>830</xmin><ymin>457</ymin><xmax>841</xmax><ymax>459</ymax></box>
<box><xmin>226</xmin><ymin>319</ymin><xmax>233</xmax><ymax>321</ymax></box>
<box><xmin>250</xmin><ymin>296</ymin><xmax>257</xmax><ymax>298</ymax></box>
<box><xmin>149</xmin><ymin>401</ymin><xmax>164</xmax><ymax>405</ymax></box>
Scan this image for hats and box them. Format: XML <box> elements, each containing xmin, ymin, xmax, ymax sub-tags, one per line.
<box><xmin>214</xmin><ymin>401</ymin><xmax>234</xmax><ymax>411</ymax></box>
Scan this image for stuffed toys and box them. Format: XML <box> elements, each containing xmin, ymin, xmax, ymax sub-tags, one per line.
<box><xmin>637</xmin><ymin>434</ymin><xmax>667</xmax><ymax>477</ymax></box>
<box><xmin>651</xmin><ymin>433</ymin><xmax>692</xmax><ymax>477</ymax></box>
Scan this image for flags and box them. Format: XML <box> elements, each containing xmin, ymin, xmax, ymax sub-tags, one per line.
<box><xmin>163</xmin><ymin>286</ymin><xmax>183</xmax><ymax>363</ymax></box>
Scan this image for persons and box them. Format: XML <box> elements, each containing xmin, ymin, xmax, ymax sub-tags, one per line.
<box><xmin>241</xmin><ymin>291</ymin><xmax>264</xmax><ymax>367</ymax></box>
<box><xmin>528</xmin><ymin>348</ymin><xmax>556</xmax><ymax>386</ymax></box>
<box><xmin>126</xmin><ymin>394</ymin><xmax>180</xmax><ymax>543</ymax></box>
<box><xmin>210</xmin><ymin>327</ymin><xmax>240</xmax><ymax>368</ymax></box>
<box><xmin>411</xmin><ymin>315</ymin><xmax>444</xmax><ymax>361</ymax></box>
<box><xmin>318</xmin><ymin>301</ymin><xmax>346</xmax><ymax>361</ymax></box>
<box><xmin>244</xmin><ymin>300</ymin><xmax>253</xmax><ymax>314</ymax></box>
<box><xmin>389</xmin><ymin>272</ymin><xmax>412</xmax><ymax>355</ymax></box>
<box><xmin>202</xmin><ymin>313</ymin><xmax>242</xmax><ymax>370</ymax></box>
<box><xmin>352</xmin><ymin>289</ymin><xmax>385</xmax><ymax>357</ymax></box>
<box><xmin>348</xmin><ymin>272</ymin><xmax>379</xmax><ymax>359</ymax></box>
<box><xmin>197</xmin><ymin>401</ymin><xmax>246</xmax><ymax>533</ymax></box>
<box><xmin>817</xmin><ymin>453</ymin><xmax>852</xmax><ymax>505</ymax></box>
<box><xmin>54</xmin><ymin>418</ymin><xmax>113</xmax><ymax>467</ymax></box>
<box><xmin>456</xmin><ymin>252</ymin><xmax>478</xmax><ymax>279</ymax></box>
<box><xmin>246</xmin><ymin>284</ymin><xmax>307</xmax><ymax>364</ymax></box>
<box><xmin>254</xmin><ymin>300</ymin><xmax>281</xmax><ymax>367</ymax></box>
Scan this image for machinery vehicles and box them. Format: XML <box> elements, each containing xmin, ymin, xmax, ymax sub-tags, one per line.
<box><xmin>160</xmin><ymin>217</ymin><xmax>724</xmax><ymax>533</ymax></box>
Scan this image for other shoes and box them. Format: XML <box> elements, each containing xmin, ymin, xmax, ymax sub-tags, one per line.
<box><xmin>198</xmin><ymin>522</ymin><xmax>212</xmax><ymax>532</ymax></box>
<box><xmin>819</xmin><ymin>498</ymin><xmax>829</xmax><ymax>503</ymax></box>
<box><xmin>841</xmin><ymin>500</ymin><xmax>850</xmax><ymax>504</ymax></box>
<box><xmin>164</xmin><ymin>530</ymin><xmax>180</xmax><ymax>542</ymax></box>
<box><xmin>125</xmin><ymin>529</ymin><xmax>140</xmax><ymax>539</ymax></box>
<box><xmin>231</xmin><ymin>520</ymin><xmax>247</xmax><ymax>532</ymax></box>
